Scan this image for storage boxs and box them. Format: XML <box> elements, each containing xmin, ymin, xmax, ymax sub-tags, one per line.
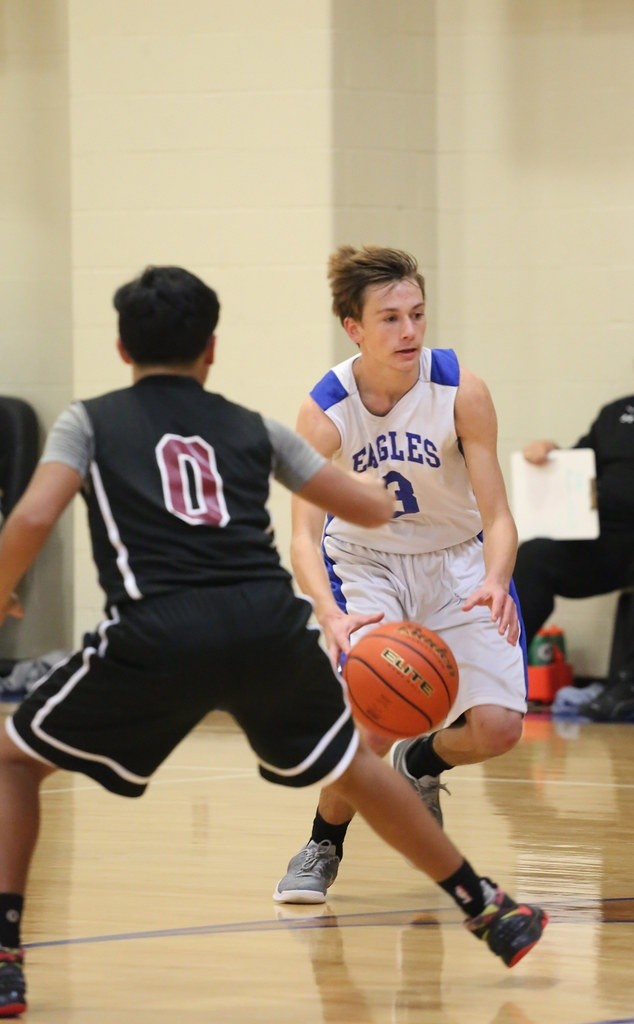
<box><xmin>524</xmin><ymin>624</ymin><xmax>575</xmax><ymax>712</ymax></box>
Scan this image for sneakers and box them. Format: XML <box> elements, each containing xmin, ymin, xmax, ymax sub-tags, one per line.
<box><xmin>463</xmin><ymin>887</ymin><xmax>550</xmax><ymax>967</ymax></box>
<box><xmin>273</xmin><ymin>839</ymin><xmax>340</xmax><ymax>905</ymax></box>
<box><xmin>575</xmin><ymin>680</ymin><xmax>633</xmax><ymax>721</ymax></box>
<box><xmin>391</xmin><ymin>735</ymin><xmax>452</xmax><ymax>834</ymax></box>
<box><xmin>0</xmin><ymin>943</ymin><xmax>27</xmax><ymax>1016</ymax></box>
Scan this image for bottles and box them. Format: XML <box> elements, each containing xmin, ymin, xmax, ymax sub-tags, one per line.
<box><xmin>527</xmin><ymin>625</ymin><xmax>566</xmax><ymax>666</ymax></box>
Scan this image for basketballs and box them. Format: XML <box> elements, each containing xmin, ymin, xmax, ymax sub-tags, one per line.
<box><xmin>343</xmin><ymin>623</ymin><xmax>460</xmax><ymax>739</ymax></box>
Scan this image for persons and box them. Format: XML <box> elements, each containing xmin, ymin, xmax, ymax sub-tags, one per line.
<box><xmin>0</xmin><ymin>266</ymin><xmax>549</xmax><ymax>1024</ymax></box>
<box><xmin>274</xmin><ymin>243</ymin><xmax>530</xmax><ymax>905</ymax></box>
<box><xmin>509</xmin><ymin>393</ymin><xmax>634</xmax><ymax>722</ymax></box>
